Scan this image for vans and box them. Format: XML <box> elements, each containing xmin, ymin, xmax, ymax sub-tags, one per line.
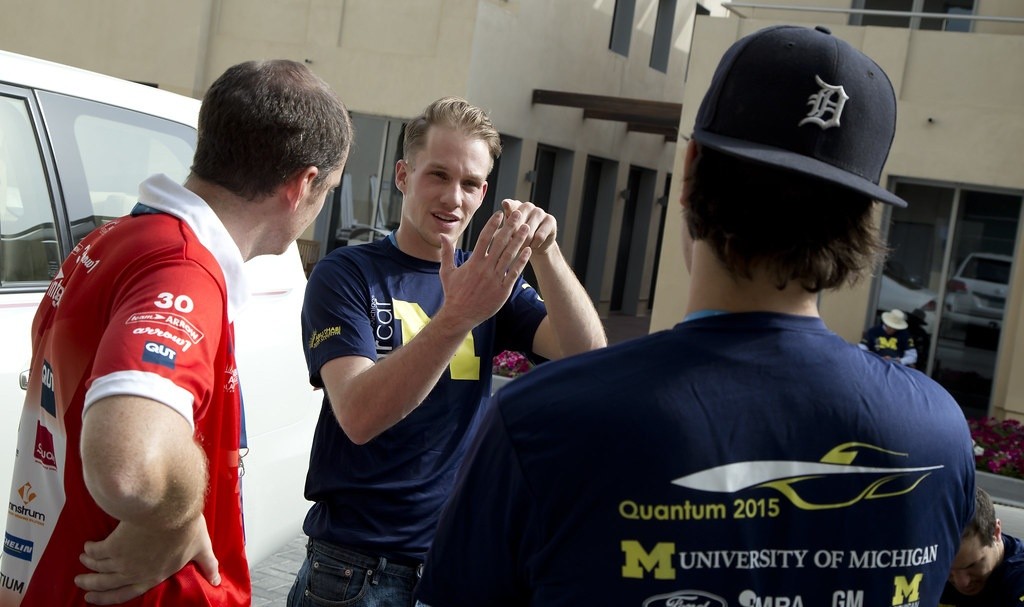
<box><xmin>0</xmin><ymin>47</ymin><xmax>323</xmax><ymax>607</ymax></box>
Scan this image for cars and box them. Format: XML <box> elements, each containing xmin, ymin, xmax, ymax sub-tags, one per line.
<box><xmin>876</xmin><ymin>259</ymin><xmax>937</xmax><ymax>338</ymax></box>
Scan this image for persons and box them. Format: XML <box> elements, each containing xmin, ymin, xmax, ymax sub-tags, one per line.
<box><xmin>0</xmin><ymin>61</ymin><xmax>352</xmax><ymax>607</ymax></box>
<box><xmin>859</xmin><ymin>309</ymin><xmax>918</xmax><ymax>369</ymax></box>
<box><xmin>413</xmin><ymin>24</ymin><xmax>975</xmax><ymax>607</ymax></box>
<box><xmin>907</xmin><ymin>310</ymin><xmax>931</xmax><ymax>371</ymax></box>
<box><xmin>941</xmin><ymin>487</ymin><xmax>1024</xmax><ymax>607</ymax></box>
<box><xmin>285</xmin><ymin>95</ymin><xmax>610</xmax><ymax>607</ymax></box>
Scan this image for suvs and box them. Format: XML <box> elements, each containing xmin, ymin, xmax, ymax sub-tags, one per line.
<box><xmin>940</xmin><ymin>251</ymin><xmax>1014</xmax><ymax>342</ymax></box>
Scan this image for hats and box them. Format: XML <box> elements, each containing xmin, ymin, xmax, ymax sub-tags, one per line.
<box><xmin>882</xmin><ymin>309</ymin><xmax>908</xmax><ymax>329</ymax></box>
<box><xmin>694</xmin><ymin>24</ymin><xmax>907</xmax><ymax>208</ymax></box>
